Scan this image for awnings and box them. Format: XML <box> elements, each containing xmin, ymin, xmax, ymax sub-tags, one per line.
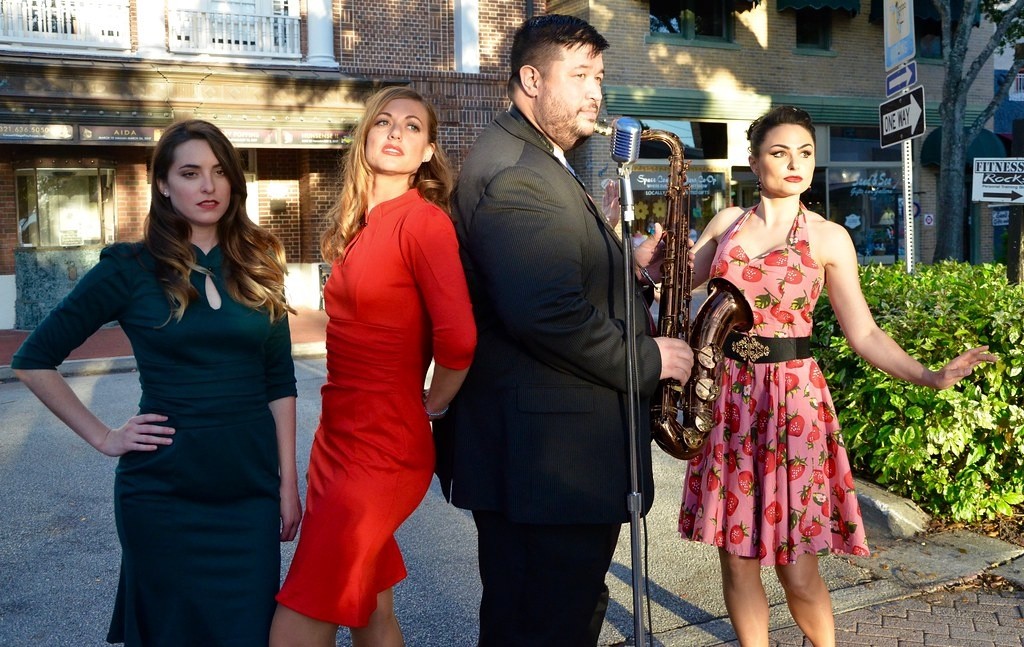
<box><xmin>629</xmin><ymin>172</ymin><xmax>725</xmax><ymax>191</ymax></box>
<box><xmin>920</xmin><ymin>126</ymin><xmax>1008</xmax><ymax>168</ymax></box>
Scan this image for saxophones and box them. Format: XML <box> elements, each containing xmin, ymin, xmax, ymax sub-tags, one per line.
<box><xmin>592</xmin><ymin>117</ymin><xmax>756</xmax><ymax>461</ymax></box>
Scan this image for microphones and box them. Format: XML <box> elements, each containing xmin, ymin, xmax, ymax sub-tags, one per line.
<box><xmin>609</xmin><ymin>117</ymin><xmax>643</xmax><ymax>235</ymax></box>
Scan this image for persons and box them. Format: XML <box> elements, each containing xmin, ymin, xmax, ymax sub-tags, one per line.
<box><xmin>10</xmin><ymin>120</ymin><xmax>302</xmax><ymax>647</ymax></box>
<box><xmin>653</xmin><ymin>104</ymin><xmax>998</xmax><ymax>647</ymax></box>
<box><xmin>431</xmin><ymin>15</ymin><xmax>696</xmax><ymax>647</ymax></box>
<box><xmin>268</xmin><ymin>87</ymin><xmax>477</xmax><ymax>647</ymax></box>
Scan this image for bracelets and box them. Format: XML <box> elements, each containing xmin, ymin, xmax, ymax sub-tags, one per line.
<box><xmin>421</xmin><ymin>389</ymin><xmax>449</xmax><ymax>415</ymax></box>
